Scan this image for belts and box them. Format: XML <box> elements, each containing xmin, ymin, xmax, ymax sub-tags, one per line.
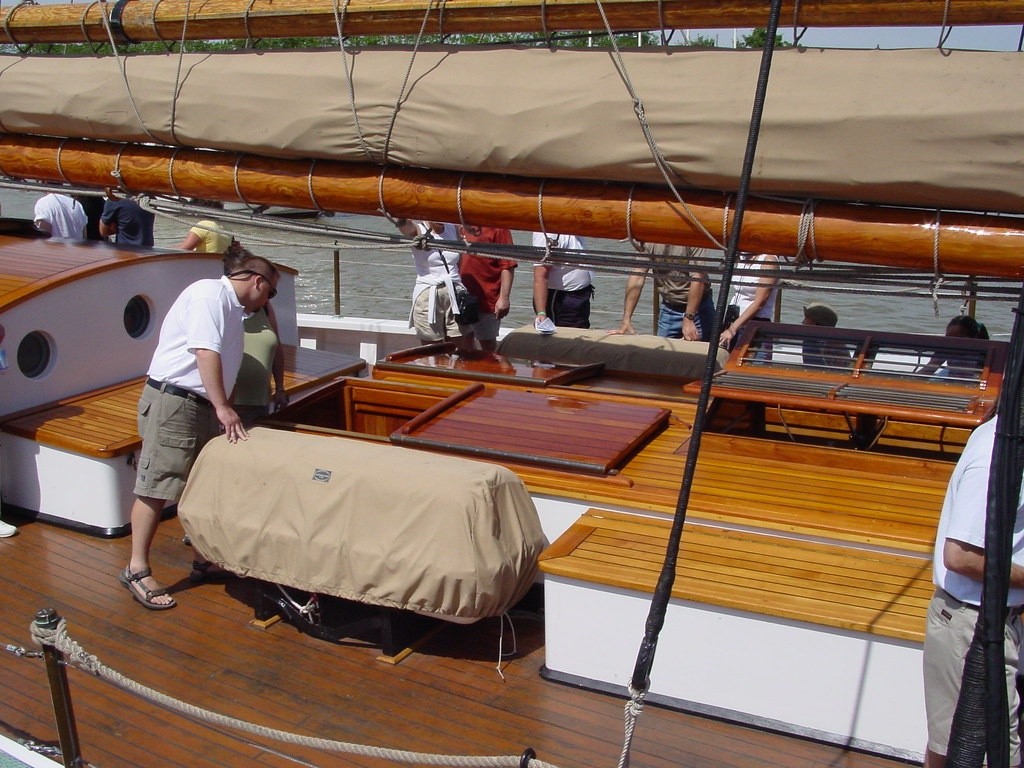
<box><xmin>663</xmin><ymin>297</ymin><xmax>712</xmax><ymax>312</ymax></box>
<box><xmin>751</xmin><ymin>317</ymin><xmax>771</xmax><ymax>322</ymax></box>
<box><xmin>146</xmin><ymin>379</ymin><xmax>214</xmax><ymax>410</ymax></box>
<box><xmin>942</xmin><ymin>588</ymin><xmax>1024</xmax><ymax>616</ymax></box>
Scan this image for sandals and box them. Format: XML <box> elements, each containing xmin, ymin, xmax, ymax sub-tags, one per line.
<box><xmin>119</xmin><ymin>562</ymin><xmax>175</xmax><ymax>609</ymax></box>
<box><xmin>190</xmin><ymin>560</ymin><xmax>235</xmax><ymax>582</ymax></box>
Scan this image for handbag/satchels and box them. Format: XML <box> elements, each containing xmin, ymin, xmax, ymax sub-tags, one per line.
<box><xmin>723</xmin><ymin>305</ymin><xmax>740</xmax><ymax>329</ymax></box>
<box><xmin>456</xmin><ymin>291</ymin><xmax>479</xmax><ymax>325</ymax></box>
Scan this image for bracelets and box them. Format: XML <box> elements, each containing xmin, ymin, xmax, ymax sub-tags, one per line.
<box><xmin>538</xmin><ymin>311</ymin><xmax>546</xmax><ymax>315</ymax></box>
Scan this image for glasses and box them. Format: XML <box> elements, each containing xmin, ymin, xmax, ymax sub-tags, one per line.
<box><xmin>252</xmin><ymin>271</ymin><xmax>278</xmax><ymax>299</ymax></box>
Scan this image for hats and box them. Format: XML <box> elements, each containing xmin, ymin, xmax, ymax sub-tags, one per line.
<box><xmin>803</xmin><ymin>305</ymin><xmax>838</xmax><ymax>327</ymax></box>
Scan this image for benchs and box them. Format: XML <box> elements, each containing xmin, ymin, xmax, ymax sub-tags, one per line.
<box><xmin>0</xmin><ymin>341</ymin><xmax>365</xmax><ymax>538</ymax></box>
<box><xmin>536</xmin><ymin>509</ymin><xmax>936</xmax><ymax>763</ymax></box>
<box><xmin>686</xmin><ymin>318</ymin><xmax>1009</xmax><ymax>448</ymax></box>
<box><xmin>373</xmin><ymin>341</ymin><xmax>723</xmax><ymax>431</ymax></box>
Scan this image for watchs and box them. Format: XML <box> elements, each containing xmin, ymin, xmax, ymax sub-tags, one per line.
<box><xmin>684</xmin><ymin>314</ymin><xmax>694</xmax><ymax>320</ymax></box>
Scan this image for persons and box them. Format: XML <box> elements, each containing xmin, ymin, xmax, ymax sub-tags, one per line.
<box><xmin>454</xmin><ymin>224</ymin><xmax>518</xmax><ymax>351</ymax></box>
<box><xmin>605</xmin><ymin>242</ymin><xmax>716</xmax><ymax>342</ymax></box>
<box><xmin>98</xmin><ymin>195</ymin><xmax>157</xmax><ymax>248</ymax></box>
<box><xmin>718</xmin><ymin>251</ymin><xmax>782</xmax><ymax>366</ymax></box>
<box><xmin>72</xmin><ymin>193</ymin><xmax>110</xmax><ymax>240</ymax></box>
<box><xmin>799</xmin><ymin>302</ymin><xmax>851</xmax><ymax>367</ymax></box>
<box><xmin>916</xmin><ymin>313</ymin><xmax>990</xmax><ymax>383</ymax></box>
<box><xmin>918</xmin><ymin>396</ymin><xmax>1024</xmax><ymax>768</ymax></box>
<box><xmin>120</xmin><ymin>255</ymin><xmax>281</xmax><ymax>610</ymax></box>
<box><xmin>30</xmin><ymin>180</ymin><xmax>90</xmax><ymax>240</ymax></box>
<box><xmin>221</xmin><ymin>246</ymin><xmax>291</xmax><ymax>426</ymax></box>
<box><xmin>167</xmin><ymin>196</ymin><xmax>236</xmax><ymax>255</ymax></box>
<box><xmin>396</xmin><ymin>219</ymin><xmax>467</xmax><ymax>347</ymax></box>
<box><xmin>531</xmin><ymin>232</ymin><xmax>595</xmax><ymax>330</ymax></box>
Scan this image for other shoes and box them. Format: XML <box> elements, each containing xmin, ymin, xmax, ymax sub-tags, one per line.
<box><xmin>0</xmin><ymin>520</ymin><xmax>16</xmax><ymax>537</ymax></box>
